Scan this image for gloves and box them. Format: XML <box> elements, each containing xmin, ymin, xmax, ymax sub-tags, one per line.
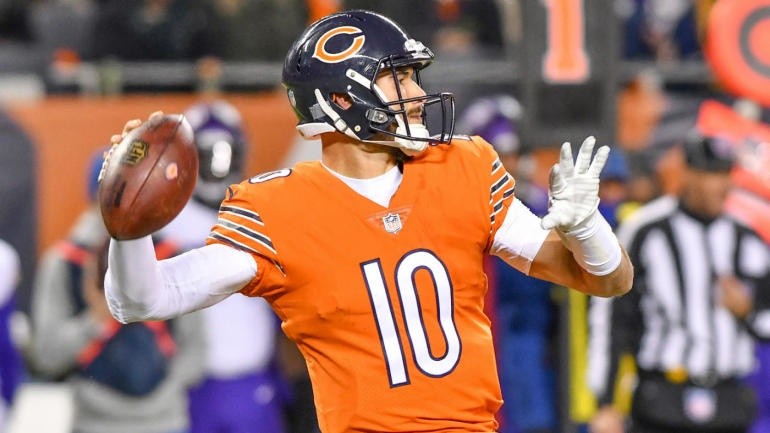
<box><xmin>540</xmin><ymin>135</ymin><xmax>622</xmax><ymax>276</ymax></box>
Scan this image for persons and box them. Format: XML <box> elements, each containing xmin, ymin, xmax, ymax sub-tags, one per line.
<box><xmin>457</xmin><ymin>96</ymin><xmax>770</xmax><ymax>433</ymax></box>
<box><xmin>98</xmin><ymin>10</ymin><xmax>635</xmax><ymax>433</ymax></box>
<box><xmin>0</xmin><ymin>101</ymin><xmax>288</xmax><ymax>433</ymax></box>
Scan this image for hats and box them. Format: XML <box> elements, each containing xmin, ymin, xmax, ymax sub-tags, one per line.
<box><xmin>683</xmin><ymin>132</ymin><xmax>737</xmax><ymax>174</ymax></box>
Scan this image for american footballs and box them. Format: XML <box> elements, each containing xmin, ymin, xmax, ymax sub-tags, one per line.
<box><xmin>99</xmin><ymin>115</ymin><xmax>199</xmax><ymax>242</ymax></box>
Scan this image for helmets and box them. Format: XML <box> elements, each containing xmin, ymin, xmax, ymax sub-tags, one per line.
<box><xmin>457</xmin><ymin>92</ymin><xmax>526</xmax><ymax>153</ymax></box>
<box><xmin>180</xmin><ymin>100</ymin><xmax>243</xmax><ymax>151</ymax></box>
<box><xmin>281</xmin><ymin>9</ymin><xmax>433</xmax><ymax>139</ymax></box>
